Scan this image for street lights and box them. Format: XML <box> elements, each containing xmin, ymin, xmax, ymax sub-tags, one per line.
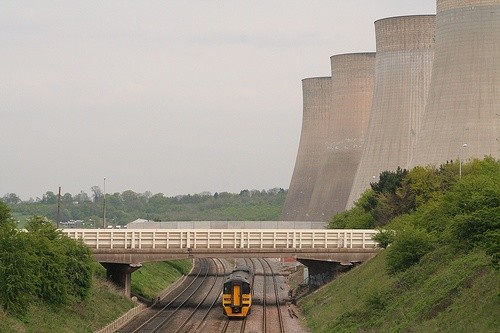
<box><xmin>102</xmin><ymin>177</ymin><xmax>106</xmax><ymax>228</ymax></box>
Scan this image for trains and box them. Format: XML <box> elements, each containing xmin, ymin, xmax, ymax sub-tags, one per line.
<box><xmin>222</xmin><ymin>265</ymin><xmax>254</xmax><ymax>319</ymax></box>
